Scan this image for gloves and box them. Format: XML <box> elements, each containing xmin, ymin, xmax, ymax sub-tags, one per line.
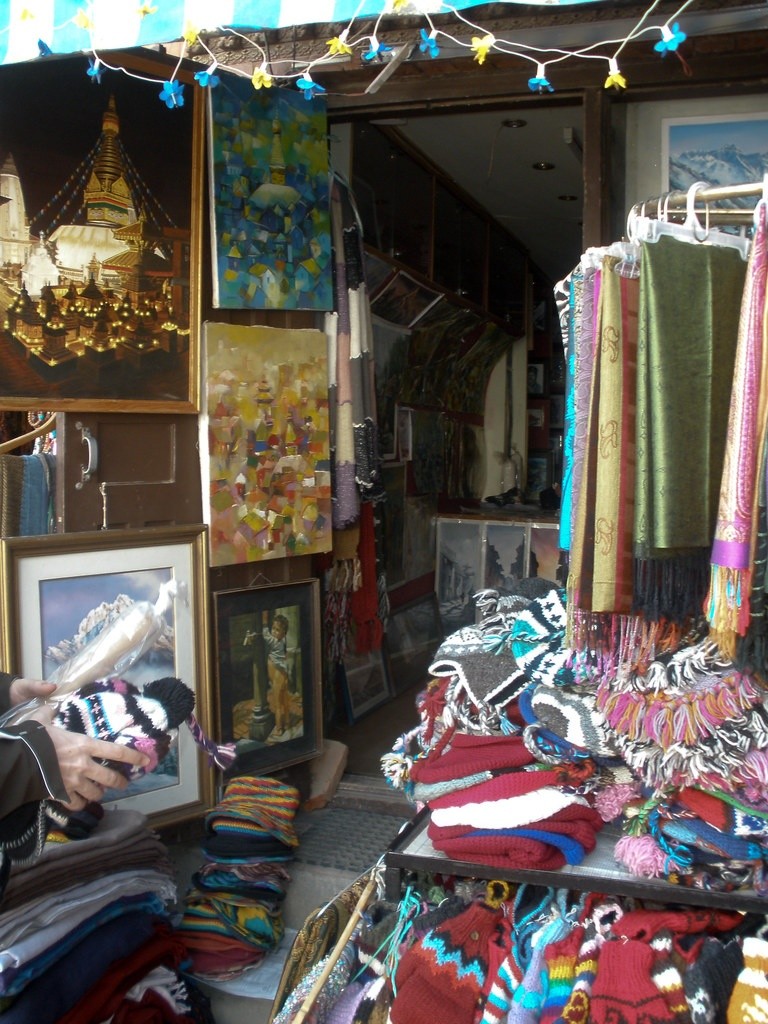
<box><xmin>357</xmin><ymin>878</ymin><xmax>768</xmax><ymax>1024</ymax></box>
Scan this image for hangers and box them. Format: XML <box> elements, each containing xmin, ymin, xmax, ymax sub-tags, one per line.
<box><xmin>754</xmin><ymin>173</ymin><xmax>768</xmax><ymax>223</ymax></box>
<box><xmin>581</xmin><ymin>181</ymin><xmax>752</xmax><ymax>272</ymax></box>
<box><xmin>0</xmin><ymin>412</ymin><xmax>57</xmax><ymax>454</ymax></box>
<box><xmin>629</xmin><ymin>618</ymin><xmax>720</xmax><ymax>703</ymax></box>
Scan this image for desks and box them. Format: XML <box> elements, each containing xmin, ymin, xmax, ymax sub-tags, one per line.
<box><xmin>435</xmin><ymin>510</ymin><xmax>568</xmax><ymax>643</ymax></box>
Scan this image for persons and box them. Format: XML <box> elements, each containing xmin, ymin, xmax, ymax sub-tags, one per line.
<box><xmin>0</xmin><ymin>670</ymin><xmax>152</xmax><ymax>877</ymax></box>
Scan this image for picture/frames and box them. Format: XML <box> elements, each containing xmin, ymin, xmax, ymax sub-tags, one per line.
<box><xmin>339</xmin><ymin>651</ymin><xmax>391</xmax><ymax>726</ymax></box>
<box><xmin>521</xmin><ymin>450</ymin><xmax>554</xmax><ymax>504</ymax></box>
<box><xmin>210</xmin><ymin>577</ymin><xmax>324</xmax><ymax>785</ymax></box>
<box><xmin>0</xmin><ymin>522</ymin><xmax>216</xmax><ymax>835</ymax></box>
<box><xmin>0</xmin><ymin>50</ymin><xmax>206</xmax><ymax>415</ymax></box>
<box><xmin>527</xmin><ymin>295</ymin><xmax>565</xmax><ymax>468</ymax></box>
<box><xmin>382</xmin><ymin>590</ymin><xmax>445</xmax><ymax>702</ymax></box>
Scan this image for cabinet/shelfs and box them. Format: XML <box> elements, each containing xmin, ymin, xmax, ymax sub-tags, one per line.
<box><xmin>328</xmin><ymin>115</ymin><xmax>527</xmax><ymax>340</ymax></box>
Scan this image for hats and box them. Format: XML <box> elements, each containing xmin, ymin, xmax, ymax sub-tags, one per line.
<box><xmin>380</xmin><ymin>579</ymin><xmax>768</xmax><ymax>896</ymax></box>
<box><xmin>176</xmin><ymin>775</ymin><xmax>300</xmax><ymax>982</ymax></box>
<box><xmin>0</xmin><ymin>677</ymin><xmax>236</xmax><ymax>867</ymax></box>
